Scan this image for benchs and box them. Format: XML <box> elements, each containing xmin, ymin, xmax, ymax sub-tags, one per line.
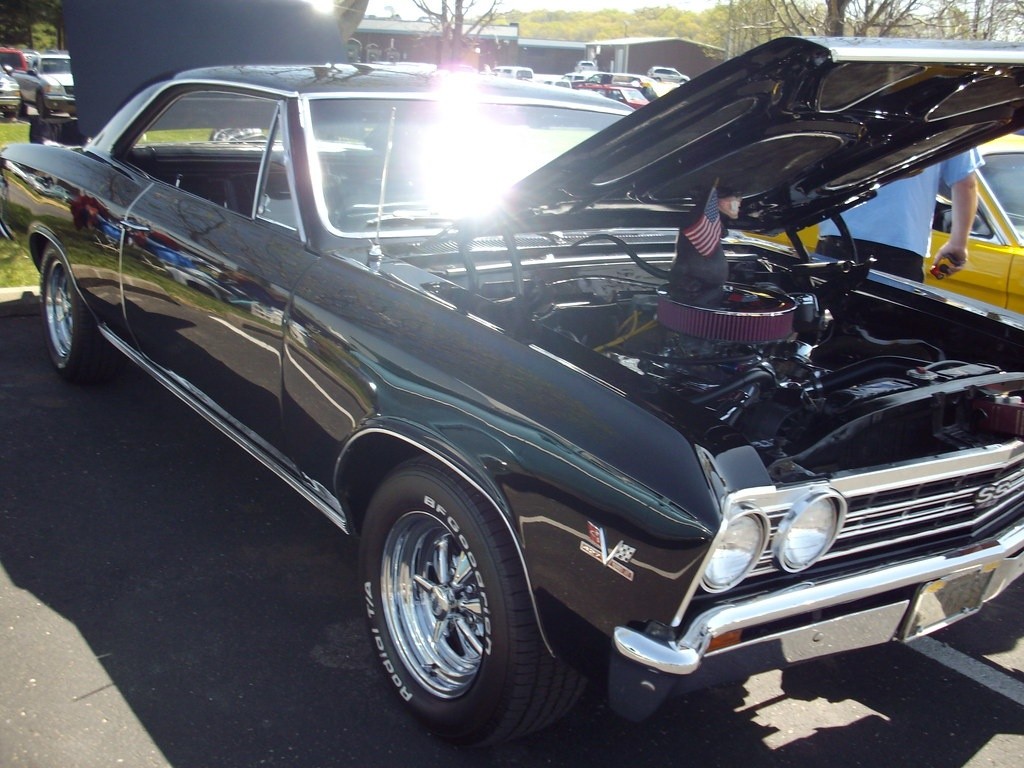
<box><xmin>148</xmin><ymin>155</ymin><xmax>407</xmax><ymax>218</ymax></box>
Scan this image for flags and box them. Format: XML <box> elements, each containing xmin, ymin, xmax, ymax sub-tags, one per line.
<box><xmin>685</xmin><ymin>187</ymin><xmax>722</xmax><ymax>258</ymax></box>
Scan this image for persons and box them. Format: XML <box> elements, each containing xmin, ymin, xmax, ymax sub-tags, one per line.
<box><xmin>815</xmin><ymin>145</ymin><xmax>986</xmax><ymax>284</ymax></box>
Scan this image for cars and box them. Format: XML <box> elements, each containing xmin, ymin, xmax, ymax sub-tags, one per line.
<box><xmin>0</xmin><ymin>1</ymin><xmax>1024</xmax><ymax>748</ymax></box>
<box><xmin>0</xmin><ymin>48</ymin><xmax>75</xmax><ymax>120</ymax></box>
<box><xmin>495</xmin><ymin>60</ymin><xmax>692</xmax><ymax>110</ymax></box>
<box><xmin>732</xmin><ymin>132</ymin><xmax>1024</xmax><ymax>316</ymax></box>
